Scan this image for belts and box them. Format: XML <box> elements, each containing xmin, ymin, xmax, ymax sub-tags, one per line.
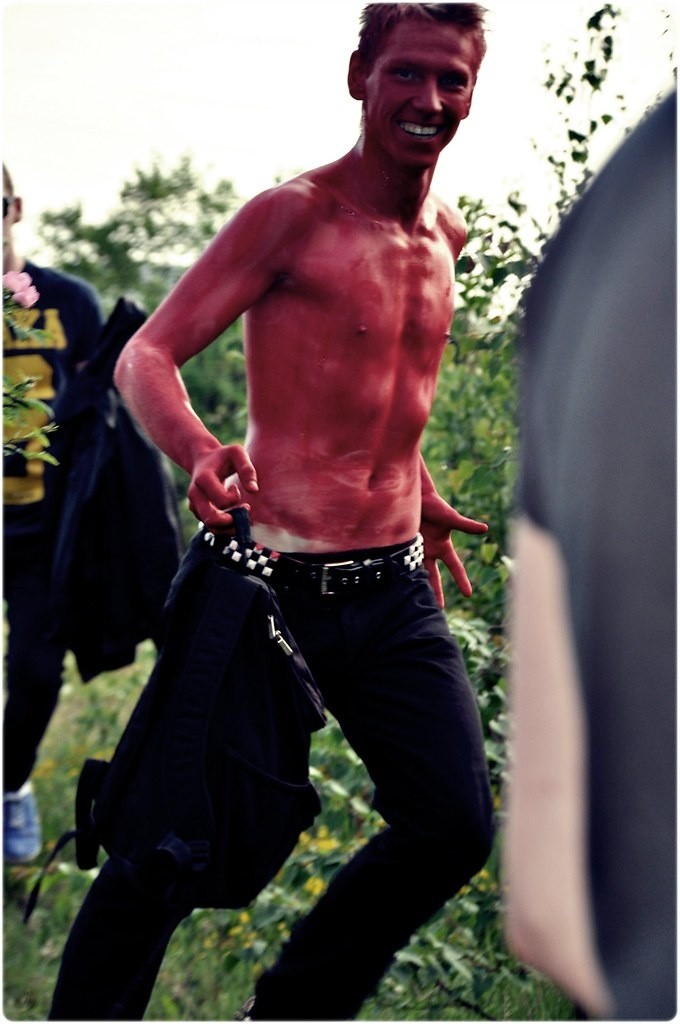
<box><xmin>194</xmin><ymin>519</ymin><xmax>430</xmax><ymax>600</ymax></box>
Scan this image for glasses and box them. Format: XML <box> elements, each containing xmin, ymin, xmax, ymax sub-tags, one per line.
<box><xmin>3</xmin><ymin>195</ymin><xmax>18</xmax><ymax>219</ymax></box>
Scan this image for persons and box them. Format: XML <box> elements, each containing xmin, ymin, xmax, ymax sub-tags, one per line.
<box><xmin>3</xmin><ymin>163</ymin><xmax>105</xmax><ymax>865</ymax></box>
<box><xmin>39</xmin><ymin>4</ymin><xmax>488</xmax><ymax>1018</ymax></box>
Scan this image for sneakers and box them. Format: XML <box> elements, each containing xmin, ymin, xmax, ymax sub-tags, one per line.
<box><xmin>3</xmin><ymin>782</ymin><xmax>42</xmax><ymax>864</ymax></box>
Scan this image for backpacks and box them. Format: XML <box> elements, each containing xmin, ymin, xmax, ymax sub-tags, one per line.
<box><xmin>21</xmin><ymin>520</ymin><xmax>324</xmax><ymax>929</ymax></box>
